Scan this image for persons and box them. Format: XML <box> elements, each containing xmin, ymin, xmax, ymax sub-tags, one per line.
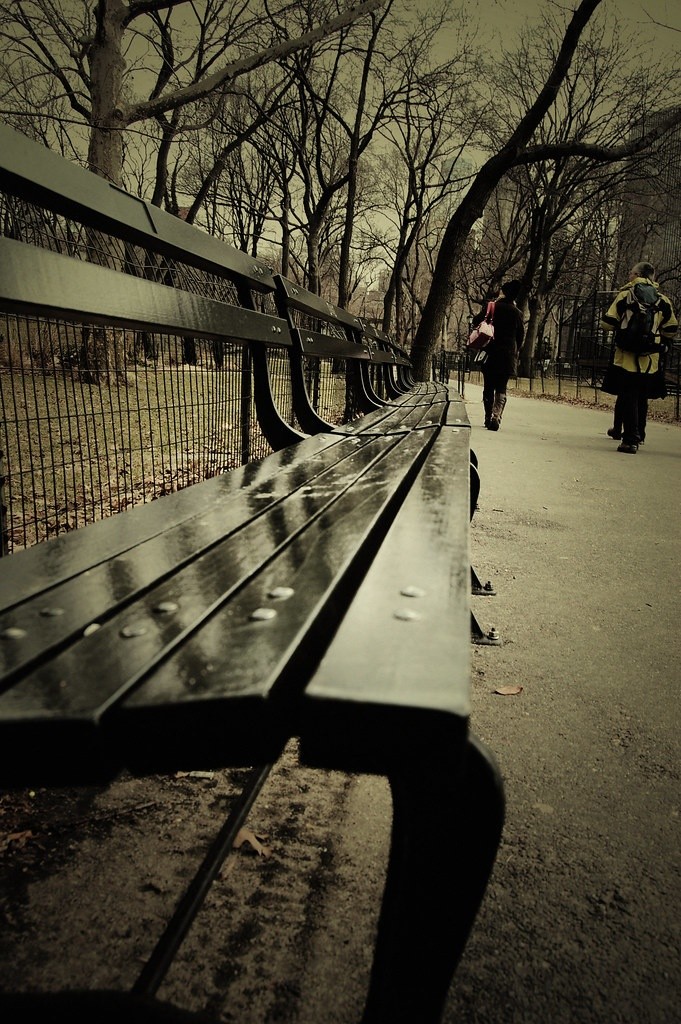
<box><xmin>473</xmin><ymin>279</ymin><xmax>525</xmax><ymax>430</ymax></box>
<box><xmin>599</xmin><ymin>262</ymin><xmax>678</xmax><ymax>454</ymax></box>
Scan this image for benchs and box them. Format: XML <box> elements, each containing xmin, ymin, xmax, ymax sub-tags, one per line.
<box><xmin>1</xmin><ymin>121</ymin><xmax>506</xmax><ymax>1023</ymax></box>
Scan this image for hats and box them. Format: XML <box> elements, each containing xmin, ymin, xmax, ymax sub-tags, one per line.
<box><xmin>501</xmin><ymin>279</ymin><xmax>524</xmax><ymax>300</ymax></box>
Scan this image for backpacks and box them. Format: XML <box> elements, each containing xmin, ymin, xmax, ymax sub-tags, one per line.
<box><xmin>615</xmin><ymin>285</ymin><xmax>669</xmax><ymax>357</ymax></box>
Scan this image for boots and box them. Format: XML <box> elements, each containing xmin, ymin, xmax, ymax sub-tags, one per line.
<box><xmin>483</xmin><ymin>391</ymin><xmax>494</xmax><ymax>427</ymax></box>
<box><xmin>488</xmin><ymin>393</ymin><xmax>507</xmax><ymax>430</ymax></box>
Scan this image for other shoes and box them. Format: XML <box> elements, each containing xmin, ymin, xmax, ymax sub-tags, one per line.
<box><xmin>638</xmin><ymin>432</ymin><xmax>646</xmax><ymax>445</ymax></box>
<box><xmin>608</xmin><ymin>427</ymin><xmax>622</xmax><ymax>440</ymax></box>
<box><xmin>617</xmin><ymin>441</ymin><xmax>638</xmax><ymax>453</ymax></box>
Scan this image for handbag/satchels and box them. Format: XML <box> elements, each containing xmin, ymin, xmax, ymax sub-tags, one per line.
<box><xmin>473</xmin><ymin>349</ymin><xmax>489</xmax><ymax>367</ymax></box>
<box><xmin>466</xmin><ymin>301</ymin><xmax>495</xmax><ymax>352</ymax></box>
